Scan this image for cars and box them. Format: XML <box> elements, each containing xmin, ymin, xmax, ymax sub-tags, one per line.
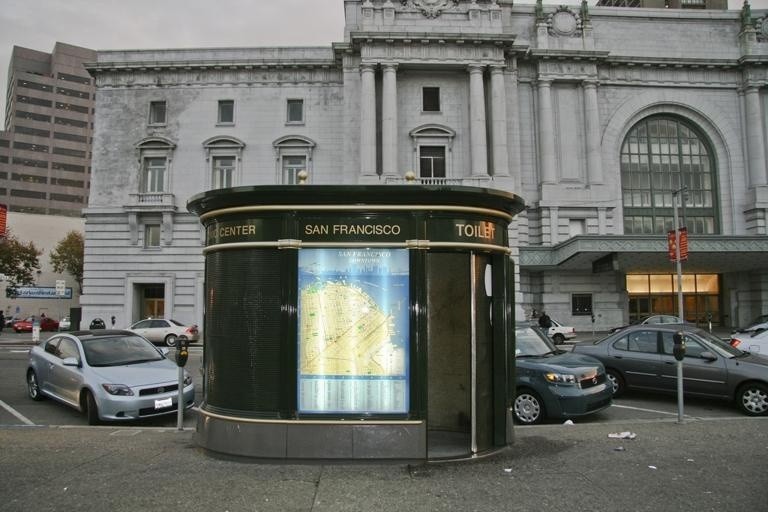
<box><xmin>512</xmin><ymin>312</ymin><xmax>768</xmax><ymax>423</ymax></box>
<box><xmin>5</xmin><ymin>314</ymin><xmax>71</xmax><ymax>332</ymax></box>
<box><xmin>124</xmin><ymin>317</ymin><xmax>199</xmax><ymax>348</ymax></box>
<box><xmin>23</xmin><ymin>330</ymin><xmax>196</xmax><ymax>425</ymax></box>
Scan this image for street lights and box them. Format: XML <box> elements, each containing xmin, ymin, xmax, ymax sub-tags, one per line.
<box><xmin>671</xmin><ymin>184</ymin><xmax>693</xmax><ymax>325</ymax></box>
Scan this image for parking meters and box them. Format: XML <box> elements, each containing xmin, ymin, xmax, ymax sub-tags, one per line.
<box><xmin>169</xmin><ymin>338</ymin><xmax>187</xmax><ymax>430</ymax></box>
<box><xmin>590</xmin><ymin>313</ymin><xmax>598</xmax><ymax>338</ymax></box>
<box><xmin>670</xmin><ymin>329</ymin><xmax>687</xmax><ymax>417</ymax></box>
<box><xmin>706</xmin><ymin>312</ymin><xmax>713</xmax><ymax>335</ymax></box>
<box><xmin>110</xmin><ymin>314</ymin><xmax>117</xmax><ymax>330</ymax></box>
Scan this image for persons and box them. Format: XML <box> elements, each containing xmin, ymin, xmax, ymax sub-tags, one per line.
<box><xmin>539</xmin><ymin>311</ymin><xmax>551</xmax><ymax>336</ymax></box>
<box><xmin>0</xmin><ymin>311</ymin><xmax>5</xmax><ymax>331</ymax></box>
